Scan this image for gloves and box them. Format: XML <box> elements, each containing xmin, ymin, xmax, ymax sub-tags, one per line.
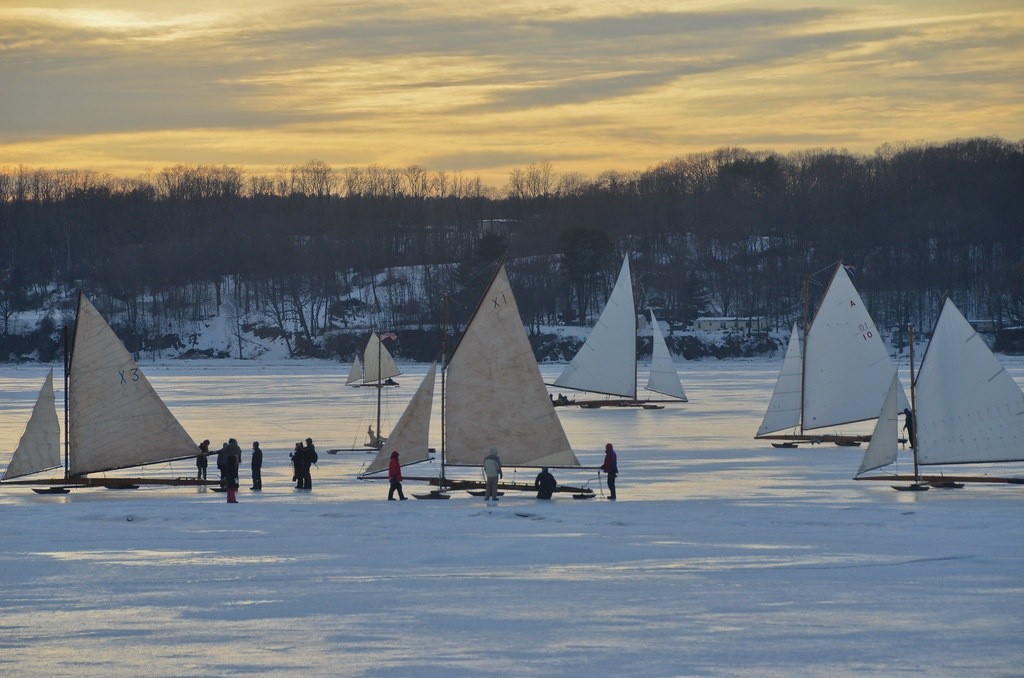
<box><xmin>500</xmin><ymin>473</ymin><xmax>502</xmax><ymax>478</ymax></box>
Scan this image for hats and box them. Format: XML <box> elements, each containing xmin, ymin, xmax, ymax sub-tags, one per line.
<box><xmin>390</xmin><ymin>452</ymin><xmax>399</xmax><ymax>459</ymax></box>
<box><xmin>490</xmin><ymin>448</ymin><xmax>496</xmax><ymax>455</ymax></box>
<box><xmin>306</xmin><ymin>438</ymin><xmax>313</xmax><ymax>442</ymax></box>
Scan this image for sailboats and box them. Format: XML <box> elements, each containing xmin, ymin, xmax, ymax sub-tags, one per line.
<box><xmin>539</xmin><ymin>250</ymin><xmax>688</xmax><ymax>410</ymax></box>
<box><xmin>343</xmin><ymin>331</ymin><xmax>405</xmax><ymax>389</ymax></box>
<box><xmin>0</xmin><ymin>292</ymin><xmax>229</xmax><ymax>495</ymax></box>
<box><xmin>850</xmin><ymin>293</ymin><xmax>1024</xmax><ymax>496</ymax></box>
<box><xmin>355</xmin><ymin>261</ymin><xmax>599</xmax><ymax>505</ymax></box>
<box><xmin>324</xmin><ymin>331</ymin><xmax>437</xmax><ymax>457</ymax></box>
<box><xmin>754</xmin><ymin>264</ymin><xmax>915</xmax><ymax>451</ymax></box>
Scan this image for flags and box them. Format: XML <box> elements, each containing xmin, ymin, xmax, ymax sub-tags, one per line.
<box><xmin>844</xmin><ymin>262</ymin><xmax>857</xmax><ymax>276</ymax></box>
<box><xmin>380</xmin><ymin>333</ymin><xmax>396</xmax><ymax>341</ymax></box>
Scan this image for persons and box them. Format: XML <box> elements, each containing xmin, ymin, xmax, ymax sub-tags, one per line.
<box><xmin>197</xmin><ymin>439</ymin><xmax>210</xmax><ymax>479</ymax></box>
<box><xmin>290</xmin><ymin>438</ymin><xmax>315</xmax><ymax>489</ymax></box>
<box><xmin>388</xmin><ymin>452</ymin><xmax>408</xmax><ymax>501</ymax></box>
<box><xmin>903</xmin><ymin>409</ymin><xmax>914</xmax><ymax>448</ymax></box>
<box><xmin>535</xmin><ymin>468</ymin><xmax>557</xmax><ymax>499</ymax></box>
<box><xmin>484</xmin><ymin>448</ymin><xmax>503</xmax><ymax>501</ymax></box>
<box><xmin>250</xmin><ymin>442</ymin><xmax>262</xmax><ymax>490</ymax></box>
<box><xmin>558</xmin><ymin>393</ymin><xmax>564</xmax><ymax>399</ymax></box>
<box><xmin>602</xmin><ymin>443</ymin><xmax>619</xmax><ymax>499</ymax></box>
<box><xmin>387</xmin><ymin>377</ymin><xmax>393</xmax><ymax>384</ymax></box>
<box><xmin>225</xmin><ymin>455</ymin><xmax>238</xmax><ymax>503</ymax></box>
<box><xmin>216</xmin><ymin>438</ymin><xmax>242</xmax><ymax>489</ymax></box>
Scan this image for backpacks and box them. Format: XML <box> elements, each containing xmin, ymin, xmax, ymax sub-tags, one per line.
<box><xmin>311</xmin><ymin>450</ymin><xmax>318</xmax><ymax>463</ymax></box>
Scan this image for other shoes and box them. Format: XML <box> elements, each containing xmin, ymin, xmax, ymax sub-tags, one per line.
<box><xmin>302</xmin><ymin>487</ymin><xmax>312</xmax><ymax>489</ymax></box>
<box><xmin>401</xmin><ymin>497</ymin><xmax>408</xmax><ymax>500</ymax></box>
<box><xmin>389</xmin><ymin>499</ymin><xmax>396</xmax><ymax>500</ymax></box>
<box><xmin>228</xmin><ymin>501</ymin><xmax>238</xmax><ymax>503</ymax></box>
<box><xmin>492</xmin><ymin>498</ymin><xmax>499</xmax><ymax>500</ymax></box>
<box><xmin>607</xmin><ymin>496</ymin><xmax>616</xmax><ymax>499</ymax></box>
<box><xmin>484</xmin><ymin>498</ymin><xmax>489</xmax><ymax>501</ymax></box>
<box><xmin>250</xmin><ymin>487</ymin><xmax>261</xmax><ymax>490</ymax></box>
<box><xmin>295</xmin><ymin>486</ymin><xmax>302</xmax><ymax>488</ymax></box>
